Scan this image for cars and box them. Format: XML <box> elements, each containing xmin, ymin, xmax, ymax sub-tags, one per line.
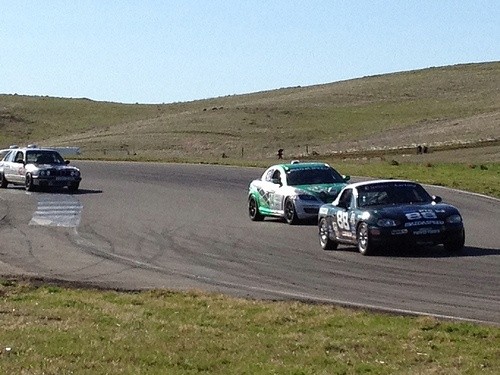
<box><xmin>248</xmin><ymin>162</ymin><xmax>352</xmax><ymax>224</ymax></box>
<box><xmin>319</xmin><ymin>178</ymin><xmax>466</xmax><ymax>255</ymax></box>
<box><xmin>3</xmin><ymin>148</ymin><xmax>81</xmax><ymax>192</ymax></box>
